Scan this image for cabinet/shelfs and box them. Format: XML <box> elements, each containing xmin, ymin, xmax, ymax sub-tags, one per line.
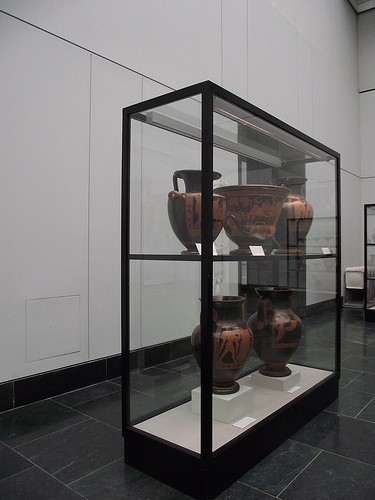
<box><xmin>364</xmin><ymin>204</ymin><xmax>375</xmax><ymax>322</ymax></box>
<box><xmin>121</xmin><ymin>79</ymin><xmax>342</xmax><ymax>500</ymax></box>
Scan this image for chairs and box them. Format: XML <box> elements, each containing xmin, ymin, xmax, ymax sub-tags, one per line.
<box><xmin>343</xmin><ymin>266</ymin><xmax>374</xmax><ymax>307</ymax></box>
